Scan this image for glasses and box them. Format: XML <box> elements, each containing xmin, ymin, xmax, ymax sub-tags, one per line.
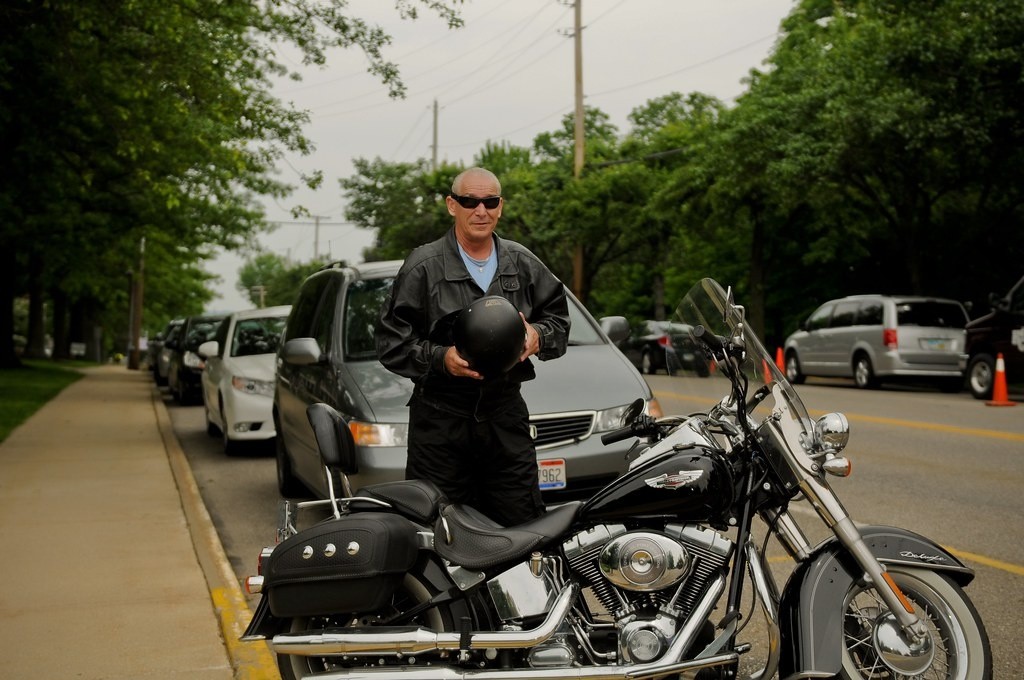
<box><xmin>451</xmin><ymin>191</ymin><xmax>500</xmax><ymax>209</ymax></box>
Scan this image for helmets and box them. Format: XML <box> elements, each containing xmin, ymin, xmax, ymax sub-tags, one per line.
<box><xmin>452</xmin><ymin>295</ymin><xmax>526</xmax><ymax>373</ymax></box>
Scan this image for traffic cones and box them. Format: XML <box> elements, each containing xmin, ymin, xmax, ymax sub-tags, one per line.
<box><xmin>776</xmin><ymin>347</ymin><xmax>785</xmax><ymax>379</ymax></box>
<box><xmin>762</xmin><ymin>358</ymin><xmax>772</xmax><ymax>383</ymax></box>
<box><xmin>986</xmin><ymin>353</ymin><xmax>1017</xmax><ymax>407</ymax></box>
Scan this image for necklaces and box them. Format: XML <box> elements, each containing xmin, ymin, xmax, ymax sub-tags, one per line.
<box><xmin>456</xmin><ymin>236</ymin><xmax>491</xmax><ymax>274</ymax></box>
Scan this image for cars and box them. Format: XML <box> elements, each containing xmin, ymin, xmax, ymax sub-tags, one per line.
<box><xmin>962</xmin><ymin>276</ymin><xmax>1023</xmax><ymax>398</ymax></box>
<box><xmin>146</xmin><ymin>315</ymin><xmax>226</xmax><ymax>403</ymax></box>
<box><xmin>618</xmin><ymin>319</ymin><xmax>710</xmax><ymax>378</ymax></box>
<box><xmin>197</xmin><ymin>304</ymin><xmax>292</xmax><ymax>457</ymax></box>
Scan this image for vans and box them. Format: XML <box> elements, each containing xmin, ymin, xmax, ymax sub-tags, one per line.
<box><xmin>783</xmin><ymin>293</ymin><xmax>974</xmax><ymax>389</ymax></box>
<box><xmin>273</xmin><ymin>258</ymin><xmax>664</xmax><ymax>513</ymax></box>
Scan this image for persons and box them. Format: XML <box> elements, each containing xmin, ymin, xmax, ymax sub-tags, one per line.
<box><xmin>373</xmin><ymin>167</ymin><xmax>572</xmax><ymax>529</ymax></box>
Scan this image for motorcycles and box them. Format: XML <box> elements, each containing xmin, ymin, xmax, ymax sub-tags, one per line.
<box><xmin>239</xmin><ymin>277</ymin><xmax>994</xmax><ymax>680</ymax></box>
